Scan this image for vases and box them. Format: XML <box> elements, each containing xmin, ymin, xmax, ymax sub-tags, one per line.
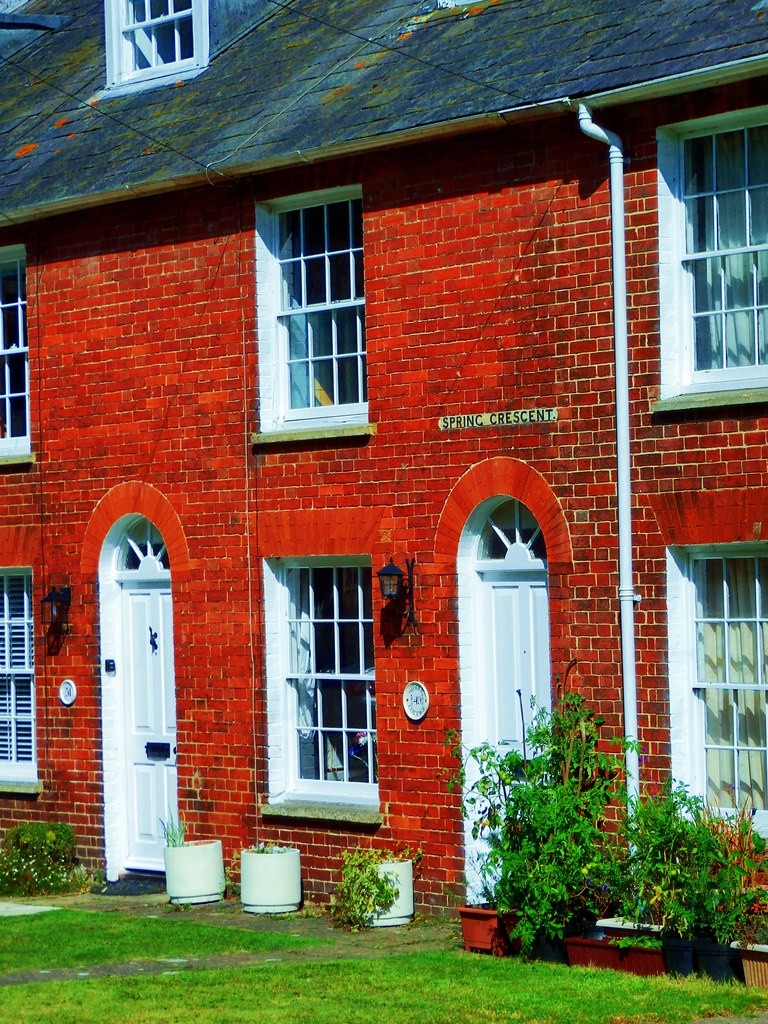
<box><xmin>240</xmin><ymin>849</ymin><xmax>306</xmax><ymax>912</ymax></box>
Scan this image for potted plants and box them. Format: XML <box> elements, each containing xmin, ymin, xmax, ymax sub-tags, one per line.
<box><xmin>332</xmin><ymin>845</ymin><xmax>422</xmax><ymax>929</ymax></box>
<box><xmin>441</xmin><ymin>692</ymin><xmax>768</xmax><ymax>987</ymax></box>
<box><xmin>158</xmin><ymin>809</ymin><xmax>224</xmax><ymax>904</ymax></box>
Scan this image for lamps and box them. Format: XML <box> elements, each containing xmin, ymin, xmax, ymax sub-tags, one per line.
<box><xmin>378</xmin><ymin>556</ymin><xmax>416</xmax><ymax>627</ymax></box>
<box><xmin>40</xmin><ymin>586</ymin><xmax>71</xmax><ymax>639</ymax></box>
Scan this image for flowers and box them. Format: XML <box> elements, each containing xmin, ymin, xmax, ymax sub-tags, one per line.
<box><xmin>249</xmin><ymin>841</ymin><xmax>290</xmax><ymax>854</ymax></box>
